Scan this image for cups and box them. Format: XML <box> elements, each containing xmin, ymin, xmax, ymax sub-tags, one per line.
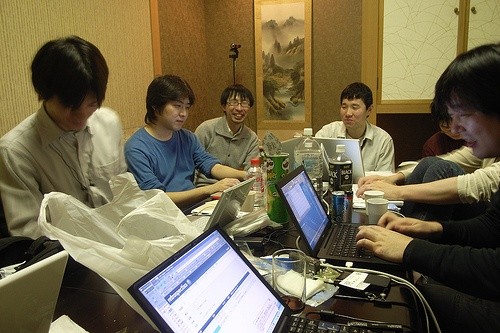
<box><xmin>240</xmin><ymin>191</ymin><xmax>256</xmax><ymax>212</ymax></box>
<box><xmin>366</xmin><ymin>198</ymin><xmax>388</xmax><ymax>224</ymax></box>
<box><xmin>272</xmin><ymin>249</ymin><xmax>307</xmax><ymax>317</ymax></box>
<box><xmin>364</xmin><ymin>190</ymin><xmax>384</xmax><ymax>216</ymax></box>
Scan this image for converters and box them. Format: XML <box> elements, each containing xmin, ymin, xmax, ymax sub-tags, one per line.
<box><xmin>235</xmin><ymin>237</ymin><xmax>267</xmax><ymax>252</ymax></box>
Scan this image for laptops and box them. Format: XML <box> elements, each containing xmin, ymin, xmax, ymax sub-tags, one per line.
<box><xmin>0</xmin><ymin>250</ymin><xmax>69</xmax><ymax>333</ymax></box>
<box><xmin>184</xmin><ymin>138</ymin><xmax>403</xmax><ymax>271</ymax></box>
<box><xmin>127</xmin><ymin>224</ymin><xmax>396</xmax><ymax>333</ymax></box>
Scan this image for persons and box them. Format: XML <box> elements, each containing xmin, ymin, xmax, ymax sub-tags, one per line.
<box><xmin>192</xmin><ymin>83</ymin><xmax>260</xmax><ymax>188</ymax></box>
<box><xmin>125</xmin><ymin>74</ymin><xmax>250</xmax><ymax>206</ymax></box>
<box><xmin>0</xmin><ymin>35</ymin><xmax>128</xmax><ymax>240</ymax></box>
<box><xmin>315</xmin><ymin>82</ymin><xmax>396</xmax><ymax>173</ymax></box>
<box><xmin>356</xmin><ymin>44</ymin><xmax>500</xmax><ymax>333</ymax></box>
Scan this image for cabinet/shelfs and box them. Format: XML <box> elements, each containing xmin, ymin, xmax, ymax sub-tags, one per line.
<box><xmin>361</xmin><ymin>0</ymin><xmax>500</xmax><ymax>111</ymax></box>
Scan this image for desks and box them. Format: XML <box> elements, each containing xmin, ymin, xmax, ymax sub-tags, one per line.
<box><xmin>48</xmin><ymin>179</ymin><xmax>410</xmax><ymax>333</ymax></box>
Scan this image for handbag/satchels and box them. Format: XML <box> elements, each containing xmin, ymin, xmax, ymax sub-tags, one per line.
<box><xmin>37</xmin><ymin>172</ymin><xmax>199</xmax><ymax>333</ymax></box>
<box><xmin>0</xmin><ymin>236</ymin><xmax>71</xmax><ymax>285</ymax></box>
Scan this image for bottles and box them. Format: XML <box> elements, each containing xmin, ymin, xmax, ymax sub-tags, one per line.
<box><xmin>327</xmin><ymin>144</ymin><xmax>353</xmax><ymax>223</ymax></box>
<box><xmin>294</xmin><ymin>128</ymin><xmax>323</xmax><ymax>205</ymax></box>
<box><xmin>261</xmin><ymin>160</ymin><xmax>268</xmax><ymax>210</ymax></box>
<box><xmin>247</xmin><ymin>158</ymin><xmax>263</xmax><ymax>207</ymax></box>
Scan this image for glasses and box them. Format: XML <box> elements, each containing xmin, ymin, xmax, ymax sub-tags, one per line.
<box><xmin>438</xmin><ymin>125</ymin><xmax>450</xmax><ymax>133</ymax></box>
<box><xmin>226</xmin><ymin>100</ymin><xmax>250</xmax><ymax>107</ymax></box>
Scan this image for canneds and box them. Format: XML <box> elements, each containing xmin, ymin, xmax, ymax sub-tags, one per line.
<box><xmin>332</xmin><ymin>191</ymin><xmax>345</xmax><ymax>216</ymax></box>
